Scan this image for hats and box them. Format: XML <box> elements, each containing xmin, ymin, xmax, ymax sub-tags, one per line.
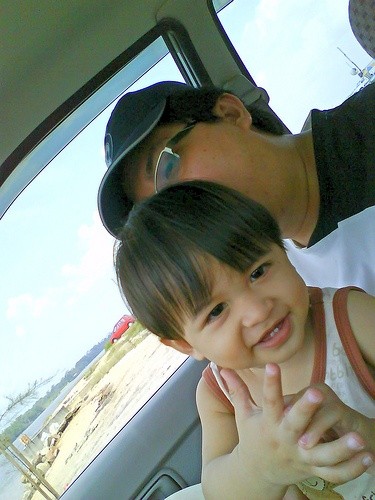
<box><xmin>96</xmin><ymin>81</ymin><xmax>197</xmax><ymax>240</ymax></box>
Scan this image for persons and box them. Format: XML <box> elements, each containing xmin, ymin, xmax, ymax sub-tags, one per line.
<box><xmin>114</xmin><ymin>179</ymin><xmax>375</xmax><ymax>500</ymax></box>
<box><xmin>97</xmin><ymin>81</ymin><xmax>375</xmax><ymax>250</ymax></box>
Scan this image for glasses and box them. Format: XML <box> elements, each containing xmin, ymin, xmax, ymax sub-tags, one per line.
<box><xmin>154</xmin><ymin>122</ymin><xmax>198</xmax><ymax>196</ymax></box>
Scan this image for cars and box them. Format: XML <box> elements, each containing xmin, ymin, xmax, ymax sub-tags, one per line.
<box><xmin>108</xmin><ymin>314</ymin><xmax>136</xmax><ymax>345</ymax></box>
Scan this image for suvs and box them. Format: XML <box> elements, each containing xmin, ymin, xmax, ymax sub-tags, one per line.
<box><xmin>352</xmin><ymin>77</ymin><xmax>370</xmax><ymax>95</ymax></box>
